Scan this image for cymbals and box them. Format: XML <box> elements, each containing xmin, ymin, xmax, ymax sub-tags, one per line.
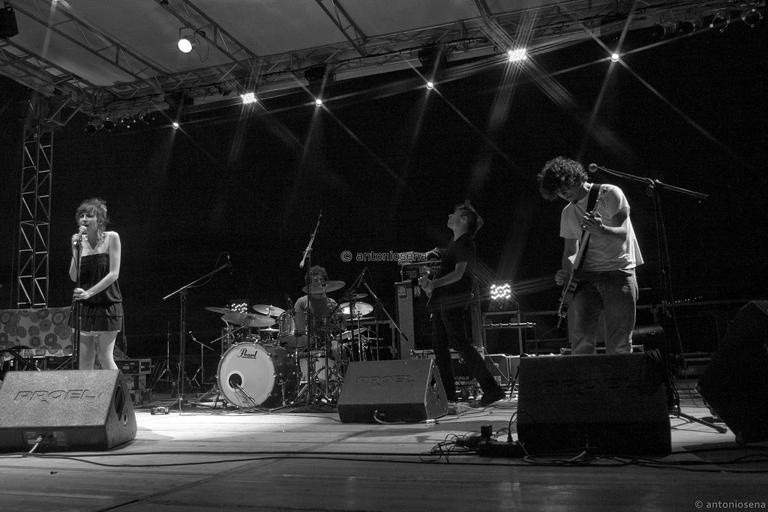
<box><xmin>339</xmin><ymin>302</ymin><xmax>374</xmax><ymax>316</ymax></box>
<box><xmin>302</xmin><ymin>280</ymin><xmax>346</xmax><ymax>294</ymax></box>
<box><xmin>204</xmin><ymin>307</ymin><xmax>234</xmax><ymax>314</ymax></box>
<box><xmin>221</xmin><ymin>312</ymin><xmax>276</xmax><ymax>327</ymax></box>
<box><xmin>333</xmin><ymin>328</ymin><xmax>367</xmax><ymax>340</ymax></box>
<box><xmin>253</xmin><ymin>304</ymin><xmax>285</xmax><ymax>317</ymax></box>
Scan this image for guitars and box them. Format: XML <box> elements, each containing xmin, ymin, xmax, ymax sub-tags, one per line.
<box><xmin>557</xmin><ymin>187</ymin><xmax>610</xmax><ymax>318</ymax></box>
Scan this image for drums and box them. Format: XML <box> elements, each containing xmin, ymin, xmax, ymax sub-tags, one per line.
<box><xmin>337</xmin><ymin>342</ymin><xmax>369</xmax><ymax>375</ymax></box>
<box><xmin>278</xmin><ymin>309</ymin><xmax>320</xmax><ymax>347</ymax></box>
<box><xmin>217</xmin><ymin>342</ymin><xmax>296</xmax><ymax>408</ymax></box>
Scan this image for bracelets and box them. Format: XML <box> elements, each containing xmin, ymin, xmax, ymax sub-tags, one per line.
<box><xmin>597</xmin><ymin>224</ymin><xmax>607</xmax><ymax>238</ymax></box>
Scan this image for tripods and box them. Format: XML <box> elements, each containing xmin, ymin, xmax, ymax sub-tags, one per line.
<box><xmin>286</xmin><ymin>209</ymin><xmax>344</xmax><ymax>407</ymax></box>
<box><xmin>461</xmin><ymin>286</ymin><xmax>522</xmax><ymax>407</ymax></box>
<box><xmin>148</xmin><ymin>264</ymin><xmax>247</xmax><ymax>411</ymax></box>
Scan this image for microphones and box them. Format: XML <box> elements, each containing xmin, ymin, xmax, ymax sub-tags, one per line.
<box><xmin>588</xmin><ymin>162</ymin><xmax>623</xmax><ymax>180</ymax></box>
<box><xmin>226</xmin><ymin>255</ymin><xmax>233</xmax><ymax>275</ymax></box>
<box><xmin>356</xmin><ymin>271</ymin><xmax>365</xmax><ymax>289</ymax></box>
<box><xmin>73</xmin><ymin>225</ymin><xmax>87</xmax><ymax>247</ymax></box>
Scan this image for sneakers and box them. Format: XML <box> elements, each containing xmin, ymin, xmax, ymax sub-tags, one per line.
<box><xmin>468</xmin><ymin>385</ymin><xmax>506</xmax><ymax>408</ymax></box>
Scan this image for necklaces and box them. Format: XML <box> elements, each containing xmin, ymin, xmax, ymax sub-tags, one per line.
<box><xmin>84</xmin><ymin>229</ymin><xmax>105</xmax><ymax>255</ymax></box>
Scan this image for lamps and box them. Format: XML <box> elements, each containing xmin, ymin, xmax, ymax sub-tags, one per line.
<box><xmin>740</xmin><ymin>3</ymin><xmax>763</xmax><ymax>28</ymax></box>
<box><xmin>675</xmin><ymin>14</ymin><xmax>702</xmax><ymax>36</ymax></box>
<box><xmin>417</xmin><ymin>47</ymin><xmax>447</xmax><ymax>90</ymax></box>
<box><xmin>304</xmin><ymin>64</ymin><xmax>336</xmax><ymax>107</ymax></box>
<box><xmin>236</xmin><ymin>78</ymin><xmax>258</xmax><ymax>106</ymax></box>
<box><xmin>709</xmin><ymin>8</ymin><xmax>730</xmax><ymax>32</ymax></box>
<box><xmin>177</xmin><ymin>25</ymin><xmax>209</xmax><ymax>54</ymax></box>
<box><xmin>164</xmin><ymin>89</ymin><xmax>194</xmax><ymax>130</ymax></box>
<box><xmin>83</xmin><ymin>96</ymin><xmax>155</xmax><ymax>136</ymax></box>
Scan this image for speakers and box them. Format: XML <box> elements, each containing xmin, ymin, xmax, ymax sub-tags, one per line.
<box><xmin>1</xmin><ymin>369</ymin><xmax>138</xmax><ymax>452</ymax></box>
<box><xmin>515</xmin><ymin>351</ymin><xmax>672</xmax><ymax>459</ymax></box>
<box><xmin>698</xmin><ymin>297</ymin><xmax>768</xmax><ymax>439</ymax></box>
<box><xmin>335</xmin><ymin>359</ymin><xmax>450</xmax><ymax>423</ymax></box>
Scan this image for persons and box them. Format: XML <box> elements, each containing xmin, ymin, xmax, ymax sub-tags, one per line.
<box><xmin>417</xmin><ymin>199</ymin><xmax>506</xmax><ymax>409</ymax></box>
<box><xmin>538</xmin><ymin>156</ymin><xmax>646</xmax><ymax>356</ymax></box>
<box><xmin>291</xmin><ymin>264</ymin><xmax>345</xmax><ymax>398</ymax></box>
<box><xmin>68</xmin><ymin>196</ymin><xmax>125</xmax><ymax>371</ymax></box>
